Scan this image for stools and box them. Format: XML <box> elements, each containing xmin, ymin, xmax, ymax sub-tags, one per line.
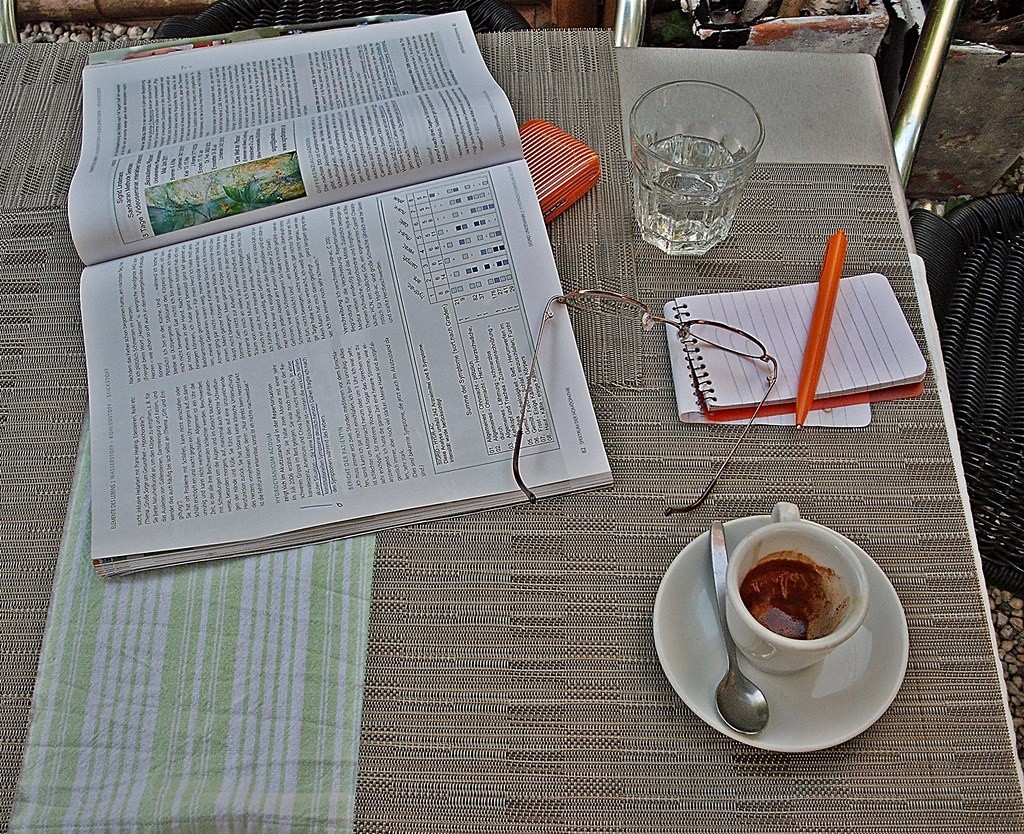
<box><xmin>913</xmin><ymin>153</ymin><xmax>1023</xmax><ymax>593</ymax></box>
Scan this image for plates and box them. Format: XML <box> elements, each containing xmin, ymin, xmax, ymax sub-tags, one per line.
<box><xmin>653</xmin><ymin>515</ymin><xmax>909</xmax><ymax>752</ymax></box>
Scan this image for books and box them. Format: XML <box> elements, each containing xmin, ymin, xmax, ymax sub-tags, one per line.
<box><xmin>65</xmin><ymin>8</ymin><xmax>613</xmax><ymax>581</ymax></box>
<box><xmin>663</xmin><ymin>270</ymin><xmax>928</xmax><ymax>430</ymax></box>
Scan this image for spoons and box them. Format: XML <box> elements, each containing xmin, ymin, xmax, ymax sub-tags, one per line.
<box><xmin>709</xmin><ymin>520</ymin><xmax>770</xmax><ymax>734</ymax></box>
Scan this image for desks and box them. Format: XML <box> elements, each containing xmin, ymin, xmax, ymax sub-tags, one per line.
<box><xmin>0</xmin><ymin>28</ymin><xmax>1024</xmax><ymax>834</ymax></box>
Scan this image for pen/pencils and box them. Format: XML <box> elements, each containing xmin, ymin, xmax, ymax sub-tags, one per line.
<box><xmin>794</xmin><ymin>227</ymin><xmax>847</xmax><ymax>431</ymax></box>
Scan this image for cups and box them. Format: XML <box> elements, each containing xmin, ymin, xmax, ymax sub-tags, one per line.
<box><xmin>628</xmin><ymin>80</ymin><xmax>766</xmax><ymax>255</ymax></box>
<box><xmin>724</xmin><ymin>501</ymin><xmax>870</xmax><ymax>672</ymax></box>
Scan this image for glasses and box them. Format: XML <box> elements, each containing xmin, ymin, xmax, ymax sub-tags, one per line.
<box><xmin>513</xmin><ymin>289</ymin><xmax>777</xmax><ymax>516</ymax></box>
